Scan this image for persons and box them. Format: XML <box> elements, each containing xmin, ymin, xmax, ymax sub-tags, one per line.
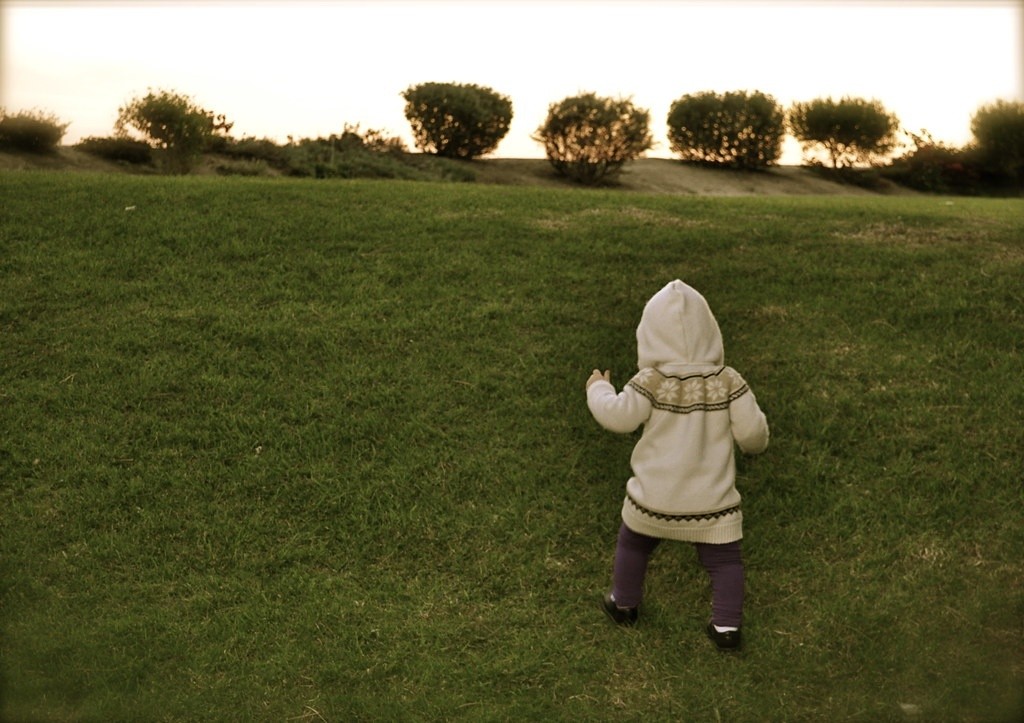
<box><xmin>586</xmin><ymin>280</ymin><xmax>770</xmax><ymax>650</ymax></box>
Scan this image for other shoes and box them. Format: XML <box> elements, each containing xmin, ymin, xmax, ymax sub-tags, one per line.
<box><xmin>601</xmin><ymin>590</ymin><xmax>639</xmax><ymax>629</ymax></box>
<box><xmin>709</xmin><ymin>623</ymin><xmax>740</xmax><ymax>651</ymax></box>
